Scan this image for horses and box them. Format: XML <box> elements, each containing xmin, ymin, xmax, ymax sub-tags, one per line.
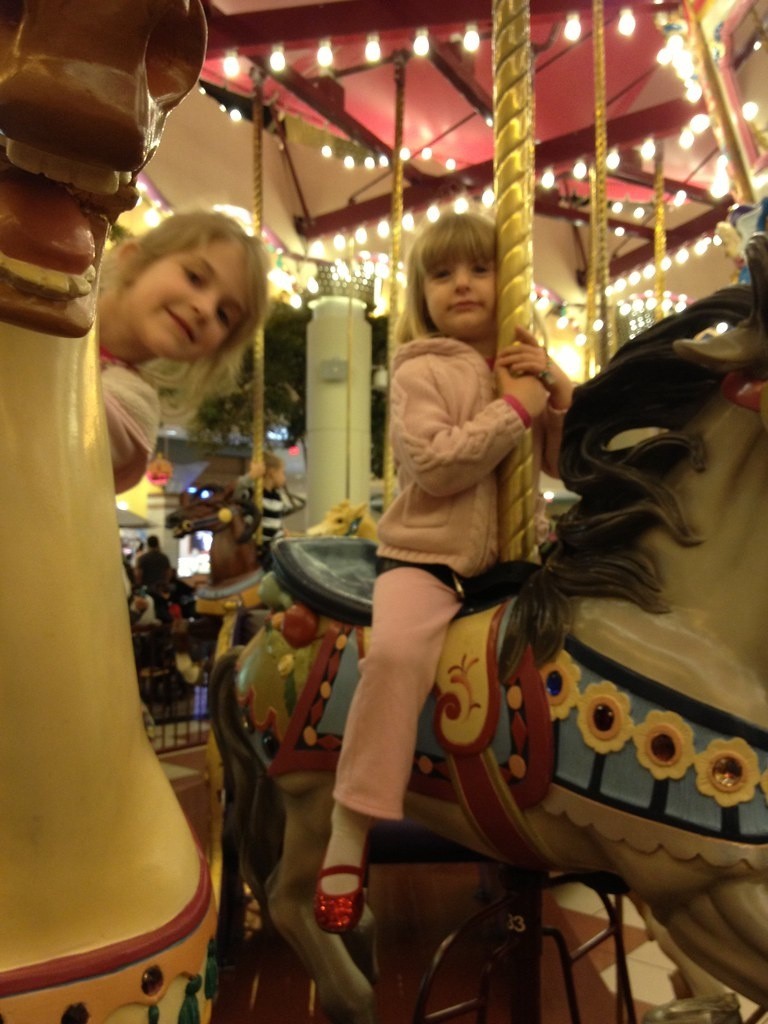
<box><xmin>0</xmin><ymin>0</ymin><xmax>768</xmax><ymax>1024</ymax></box>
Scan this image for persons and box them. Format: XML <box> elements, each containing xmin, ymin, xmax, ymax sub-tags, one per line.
<box><xmin>234</xmin><ymin>453</ymin><xmax>288</xmax><ymax>574</ymax></box>
<box><xmin>314</xmin><ymin>212</ymin><xmax>577</xmax><ymax>934</ymax></box>
<box><xmin>136</xmin><ymin>535</ymin><xmax>172</xmax><ymax>595</ymax></box>
<box><xmin>92</xmin><ymin>207</ymin><xmax>270</xmax><ymax>497</ymax></box>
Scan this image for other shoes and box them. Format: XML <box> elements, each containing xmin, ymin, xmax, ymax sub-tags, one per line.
<box><xmin>314</xmin><ymin>834</ymin><xmax>368</xmax><ymax>934</ymax></box>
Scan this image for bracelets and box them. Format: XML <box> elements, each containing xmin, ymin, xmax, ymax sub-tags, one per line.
<box><xmin>535</xmin><ymin>347</ymin><xmax>552</xmax><ymax>379</ymax></box>
